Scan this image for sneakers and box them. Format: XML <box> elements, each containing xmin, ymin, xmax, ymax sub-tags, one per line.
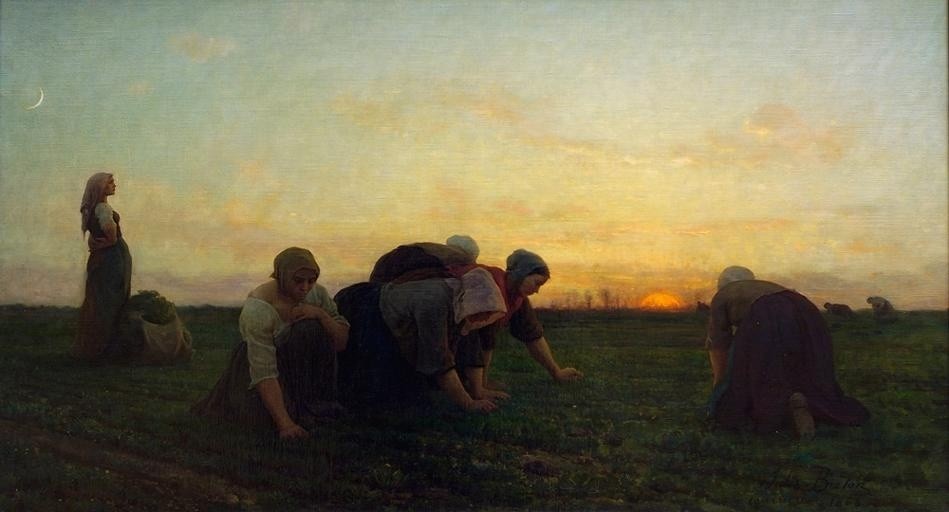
<box><xmin>789</xmin><ymin>391</ymin><xmax>818</xmax><ymax>443</ymax></box>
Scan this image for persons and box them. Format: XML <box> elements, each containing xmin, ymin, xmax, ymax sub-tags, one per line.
<box><xmin>824</xmin><ymin>303</ymin><xmax>855</xmax><ymax>320</ymax></box>
<box><xmin>389</xmin><ymin>249</ymin><xmax>582</xmax><ymax>383</ymax></box>
<box><xmin>369</xmin><ymin>235</ymin><xmax>479</xmax><ymax>283</ymax></box>
<box><xmin>696</xmin><ymin>301</ymin><xmax>711</xmax><ymax>312</ymax></box>
<box><xmin>70</xmin><ymin>173</ymin><xmax>132</xmax><ymax>361</ymax></box>
<box><xmin>333</xmin><ymin>267</ymin><xmax>511</xmax><ymax>414</ymax></box>
<box><xmin>190</xmin><ymin>246</ymin><xmax>351</xmax><ymax>440</ymax></box>
<box><xmin>705</xmin><ymin>266</ymin><xmax>880</xmax><ymax>441</ymax></box>
<box><xmin>867</xmin><ymin>296</ymin><xmax>894</xmax><ymax>316</ymax></box>
